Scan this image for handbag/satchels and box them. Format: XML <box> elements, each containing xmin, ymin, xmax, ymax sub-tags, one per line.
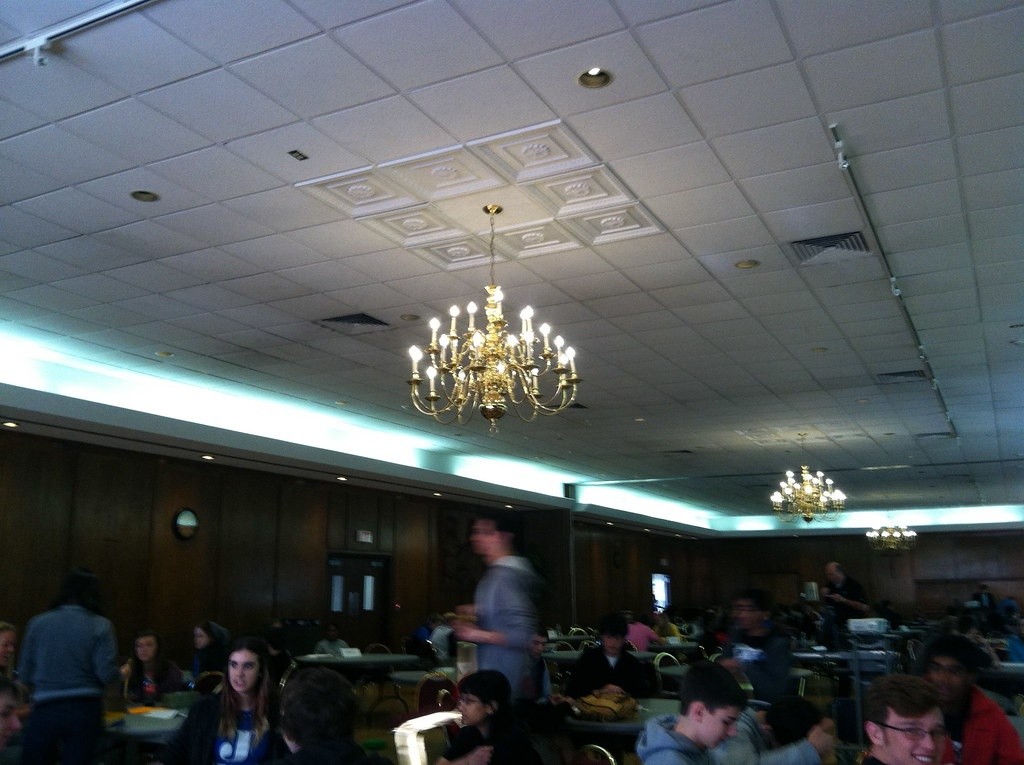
<box><xmin>580</xmin><ymin>689</ymin><xmax>639</xmax><ymax>724</ymax></box>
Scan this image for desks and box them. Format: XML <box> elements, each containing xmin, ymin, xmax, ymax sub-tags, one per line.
<box><xmin>549</xmin><ymin>636</ymin><xmax>594</xmax><ymax>647</ymax></box>
<box><xmin>541</xmin><ymin>649</ymin><xmax>653</xmax><ymax>665</ymax></box>
<box><xmin>112</xmin><ymin>709</ymin><xmax>189</xmax><ymax>743</ymax></box>
<box><xmin>980</xmin><ymin>662</ymin><xmax>1024</xmax><ymax>678</ymax></box>
<box><xmin>565</xmin><ymin>698</ymin><xmax>682</xmax><ymax>735</ymax></box>
<box><xmin>648</xmin><ymin>641</ymin><xmax>698</xmax><ymax>651</ymax></box>
<box><xmin>910</xmin><ymin>625</ymin><xmax>931</xmax><ymax>629</ymax></box>
<box><xmin>793</xmin><ymin>650</ymin><xmax>899</xmax><ymax>660</ymax></box>
<box><xmin>388</xmin><ymin>670</ymin><xmax>426</xmax><ymax>683</ymax></box>
<box><xmin>1007</xmin><ymin>715</ymin><xmax>1024</xmax><ymax>741</ymax></box>
<box><xmin>660</xmin><ymin>665</ymin><xmax>814</xmax><ymax>697</ymax></box>
<box><xmin>295</xmin><ymin>654</ymin><xmax>418</xmax><ymax>668</ymax></box>
<box><xmin>888</xmin><ymin>629</ymin><xmax>923</xmax><ymax>635</ymax></box>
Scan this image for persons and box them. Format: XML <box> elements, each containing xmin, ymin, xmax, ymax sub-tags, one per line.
<box><xmin>450</xmin><ymin>509</ymin><xmax>544</xmax><ymax>705</ymax></box>
<box><xmin>919</xmin><ymin>634</ymin><xmax>1024</xmax><ymax>765</ymax></box>
<box><xmin>772</xmin><ymin>602</ymin><xmax>825</xmax><ymax>637</ymax></box>
<box><xmin>121</xmin><ymin>630</ymin><xmax>184</xmax><ymax>706</ymax></box>
<box><xmin>17</xmin><ymin>568</ymin><xmax>132</xmax><ymax>765</ymax></box>
<box><xmin>158</xmin><ymin>637</ymin><xmax>288</xmax><ymax>765</ymax></box>
<box><xmin>416</xmin><ymin>611</ymin><xmax>458</xmax><ymax>665</ymax></box>
<box><xmin>526</xmin><ymin>628</ymin><xmax>552</xmax><ymax>698</ymax></box>
<box><xmin>861</xmin><ymin>675</ymin><xmax>951</xmax><ymax>765</ymax></box>
<box><xmin>816</xmin><ymin>561</ymin><xmax>871</xmax><ymax>689</ymax></box>
<box><xmin>564</xmin><ymin>614</ymin><xmax>651</xmax><ymax>699</ymax></box>
<box><xmin>710</xmin><ymin>695</ymin><xmax>833</xmax><ymax>765</ymax></box>
<box><xmin>952</xmin><ymin>584</ymin><xmax>1024</xmax><ymax>663</ymax></box>
<box><xmin>719</xmin><ymin>589</ymin><xmax>788</xmax><ymax>710</ymax></box>
<box><xmin>262</xmin><ymin>617</ymin><xmax>289</xmax><ymax>669</ymax></box>
<box><xmin>626</xmin><ymin>609</ymin><xmax>728</xmax><ymax>651</ymax></box>
<box><xmin>0</xmin><ymin>621</ymin><xmax>23</xmax><ymax>752</ymax></box>
<box><xmin>636</xmin><ymin>658</ymin><xmax>749</xmax><ymax>765</ymax></box>
<box><xmin>876</xmin><ymin>599</ymin><xmax>902</xmax><ymax>629</ymax></box>
<box><xmin>271</xmin><ymin>667</ymin><xmax>393</xmax><ymax>765</ymax></box>
<box><xmin>434</xmin><ymin>669</ymin><xmax>543</xmax><ymax>765</ymax></box>
<box><xmin>193</xmin><ymin>620</ymin><xmax>230</xmax><ymax>694</ymax></box>
<box><xmin>315</xmin><ymin>624</ymin><xmax>347</xmax><ymax>655</ymax></box>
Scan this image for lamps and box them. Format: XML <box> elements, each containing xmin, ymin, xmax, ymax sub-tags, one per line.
<box><xmin>405</xmin><ymin>204</ymin><xmax>585</xmax><ymax>439</ymax></box>
<box><xmin>866</xmin><ymin>526</ymin><xmax>917</xmax><ymax>556</ymax></box>
<box><xmin>172</xmin><ymin>508</ymin><xmax>198</xmax><ymax>540</ymax></box>
<box><xmin>770</xmin><ymin>433</ymin><xmax>848</xmax><ymax>525</ymax></box>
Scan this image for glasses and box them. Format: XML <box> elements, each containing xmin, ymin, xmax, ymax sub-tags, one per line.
<box><xmin>877</xmin><ymin>720</ymin><xmax>950</xmax><ymax>745</ymax></box>
<box><xmin>455</xmin><ymin>696</ymin><xmax>484</xmax><ymax>710</ymax></box>
<box><xmin>928</xmin><ymin>662</ymin><xmax>973</xmax><ymax>681</ymax></box>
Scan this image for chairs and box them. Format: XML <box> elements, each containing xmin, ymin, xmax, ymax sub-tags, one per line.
<box><xmin>13</xmin><ymin>618</ymin><xmax>1024</xmax><ymax>765</ymax></box>
